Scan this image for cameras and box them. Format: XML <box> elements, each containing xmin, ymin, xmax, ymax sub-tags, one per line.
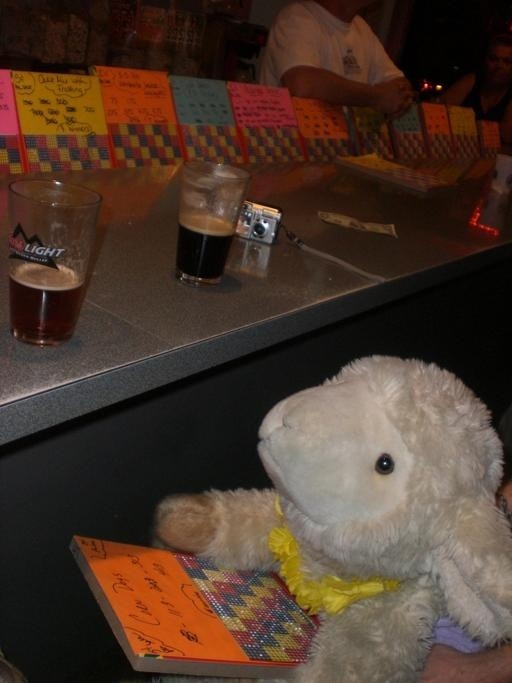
<box><xmin>236</xmin><ymin>201</ymin><xmax>282</xmax><ymax>246</ymax></box>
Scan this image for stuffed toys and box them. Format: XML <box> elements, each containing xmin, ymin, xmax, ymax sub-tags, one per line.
<box><xmin>153</xmin><ymin>353</ymin><xmax>512</xmax><ymax>683</ymax></box>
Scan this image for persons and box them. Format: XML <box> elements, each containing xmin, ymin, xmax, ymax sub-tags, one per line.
<box><xmin>421</xmin><ymin>634</ymin><xmax>512</xmax><ymax>683</ymax></box>
<box><xmin>257</xmin><ymin>1</ymin><xmax>415</xmax><ymax>116</ymax></box>
<box><xmin>435</xmin><ymin>43</ymin><xmax>512</xmax><ymax>143</ymax></box>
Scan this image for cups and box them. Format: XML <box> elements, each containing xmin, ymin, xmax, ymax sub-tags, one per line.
<box><xmin>172</xmin><ymin>156</ymin><xmax>252</xmax><ymax>289</ymax></box>
<box><xmin>5</xmin><ymin>176</ymin><xmax>103</xmax><ymax>348</ymax></box>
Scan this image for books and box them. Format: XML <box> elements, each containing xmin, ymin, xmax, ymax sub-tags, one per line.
<box><xmin>333</xmin><ymin>150</ymin><xmax>459</xmax><ymax>195</ymax></box>
<box><xmin>69</xmin><ymin>535</ymin><xmax>322</xmax><ymax>674</ymax></box>
